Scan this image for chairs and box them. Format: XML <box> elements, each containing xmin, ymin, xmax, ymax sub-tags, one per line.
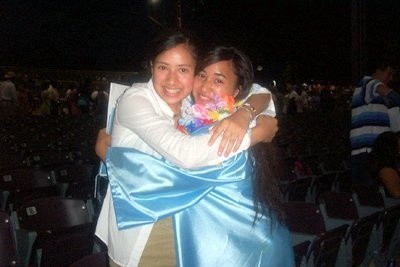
<box><xmin>1</xmin><ymin>145</ymin><xmax>110</xmax><ymax>267</ymax></box>
<box><xmin>254</xmin><ymin>128</ymin><xmax>400</xmax><ymax>267</ymax></box>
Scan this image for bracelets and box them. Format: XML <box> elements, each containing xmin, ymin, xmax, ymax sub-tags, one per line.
<box><xmin>240</xmin><ymin>106</ymin><xmax>253</xmax><ymax>122</ymax></box>
<box><xmin>242</xmin><ymin>102</ymin><xmax>258</xmax><ymax>118</ymax></box>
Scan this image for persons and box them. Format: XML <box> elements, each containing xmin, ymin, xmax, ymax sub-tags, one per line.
<box><xmin>94</xmin><ymin>45</ymin><xmax>296</xmax><ymax>267</ymax></box>
<box><xmin>94</xmin><ymin>29</ymin><xmax>280</xmax><ymax>266</ymax></box>
<box><xmin>348</xmin><ymin>60</ymin><xmax>400</xmax><ymax>199</ymax></box>
<box><xmin>241</xmin><ymin>59</ymin><xmax>361</xmax><ymax>148</ymax></box>
<box><xmin>0</xmin><ymin>67</ymin><xmax>120</xmax><ymax>125</ymax></box>
<box><xmin>366</xmin><ymin>129</ymin><xmax>400</xmax><ymax>199</ymax></box>
